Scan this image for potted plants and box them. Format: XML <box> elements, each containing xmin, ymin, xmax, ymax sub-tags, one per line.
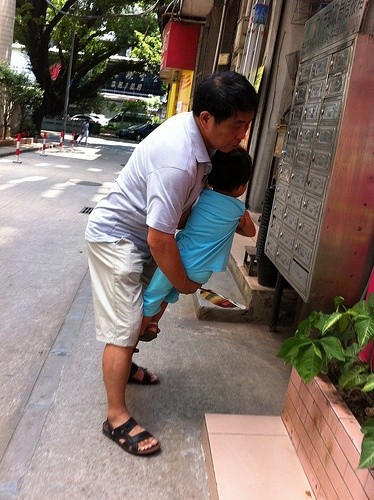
<box><xmin>276</xmin><ymin>296</ymin><xmax>374</xmax><ymax>500</ymax></box>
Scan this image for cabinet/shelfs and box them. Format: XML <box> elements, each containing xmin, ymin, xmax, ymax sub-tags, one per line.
<box><xmin>262</xmin><ymin>32</ymin><xmax>374</xmax><ymax>304</ymax></box>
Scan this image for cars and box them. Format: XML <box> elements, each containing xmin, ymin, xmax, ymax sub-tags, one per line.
<box><xmin>69</xmin><ymin>114</ymin><xmax>106</xmax><ymax>128</ymax></box>
<box><xmin>117</xmin><ymin>123</ymin><xmax>161</xmax><ymax>143</ymax></box>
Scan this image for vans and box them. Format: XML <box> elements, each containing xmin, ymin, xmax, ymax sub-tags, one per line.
<box><xmin>108</xmin><ymin>112</ymin><xmax>154</xmax><ymax>131</ymax></box>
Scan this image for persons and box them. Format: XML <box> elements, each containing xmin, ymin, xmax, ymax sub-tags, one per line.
<box><xmin>134</xmin><ymin>143</ymin><xmax>258</xmax><ymax>355</ymax></box>
<box><xmin>84</xmin><ymin>70</ymin><xmax>259</xmax><ymax>462</ymax></box>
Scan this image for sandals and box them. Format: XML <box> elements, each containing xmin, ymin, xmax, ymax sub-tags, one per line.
<box><xmin>126</xmin><ymin>362</ymin><xmax>160</xmax><ymax>385</ymax></box>
<box><xmin>139</xmin><ymin>321</ymin><xmax>160</xmax><ymax>342</ymax></box>
<box><xmin>133</xmin><ymin>347</ymin><xmax>139</xmax><ymax>353</ymax></box>
<box><xmin>102</xmin><ymin>417</ymin><xmax>160</xmax><ymax>454</ymax></box>
